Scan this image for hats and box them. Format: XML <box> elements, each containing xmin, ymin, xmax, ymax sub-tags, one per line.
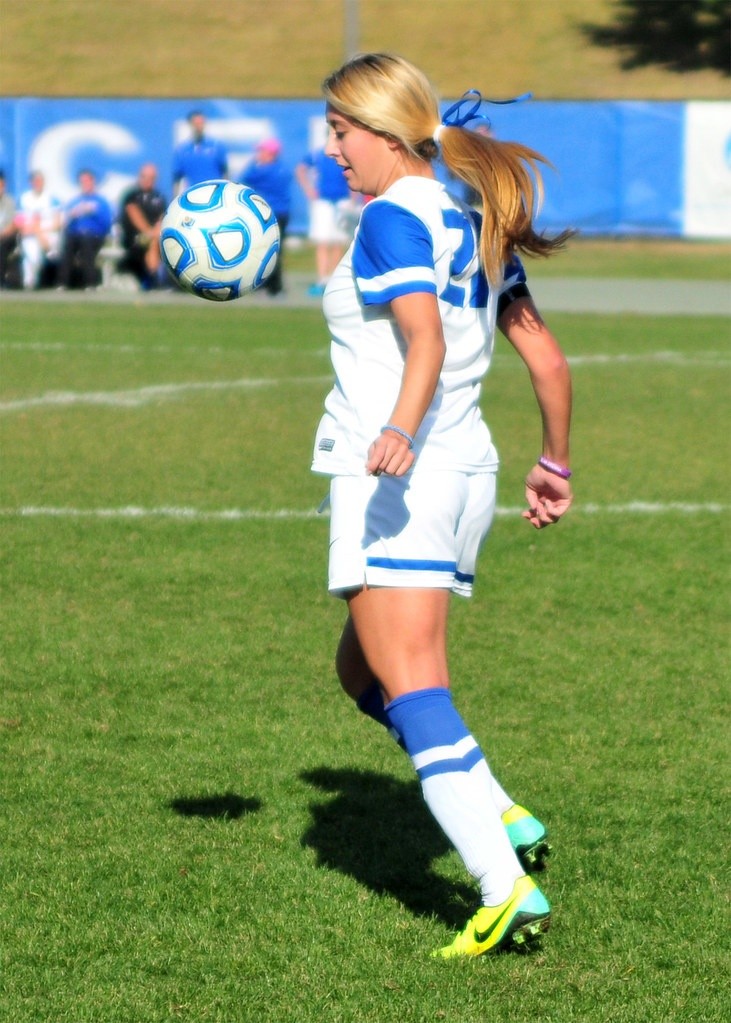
<box><xmin>255</xmin><ymin>137</ymin><xmax>279</xmax><ymax>153</ymax></box>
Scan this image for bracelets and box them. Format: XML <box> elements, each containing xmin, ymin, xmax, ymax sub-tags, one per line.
<box><xmin>537</xmin><ymin>454</ymin><xmax>572</xmax><ymax>479</ymax></box>
<box><xmin>380</xmin><ymin>423</ymin><xmax>415</xmax><ymax>449</ymax></box>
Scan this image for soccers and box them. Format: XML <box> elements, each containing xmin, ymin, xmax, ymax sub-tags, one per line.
<box><xmin>158</xmin><ymin>178</ymin><xmax>281</xmax><ymax>301</ymax></box>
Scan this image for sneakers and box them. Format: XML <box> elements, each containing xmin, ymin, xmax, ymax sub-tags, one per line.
<box><xmin>429</xmin><ymin>876</ymin><xmax>553</xmax><ymax>959</ymax></box>
<box><xmin>501</xmin><ymin>802</ymin><xmax>553</xmax><ymax>865</ymax></box>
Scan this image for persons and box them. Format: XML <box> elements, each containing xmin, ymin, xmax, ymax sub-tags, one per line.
<box><xmin>464</xmin><ymin>118</ymin><xmax>506</xmax><ymax>210</ymax></box>
<box><xmin>13</xmin><ymin>170</ymin><xmax>66</xmax><ymax>295</ymax></box>
<box><xmin>293</xmin><ymin>126</ymin><xmax>361</xmax><ymax>296</ymax></box>
<box><xmin>0</xmin><ymin>163</ymin><xmax>18</xmax><ymax>290</ymax></box>
<box><xmin>235</xmin><ymin>138</ymin><xmax>294</xmax><ymax>297</ymax></box>
<box><xmin>309</xmin><ymin>52</ymin><xmax>577</xmax><ymax>959</ymax></box>
<box><xmin>113</xmin><ymin>161</ymin><xmax>166</xmax><ymax>290</ymax></box>
<box><xmin>56</xmin><ymin>170</ymin><xmax>113</xmax><ymax>292</ymax></box>
<box><xmin>169</xmin><ymin>110</ymin><xmax>233</xmax><ymax>193</ymax></box>
